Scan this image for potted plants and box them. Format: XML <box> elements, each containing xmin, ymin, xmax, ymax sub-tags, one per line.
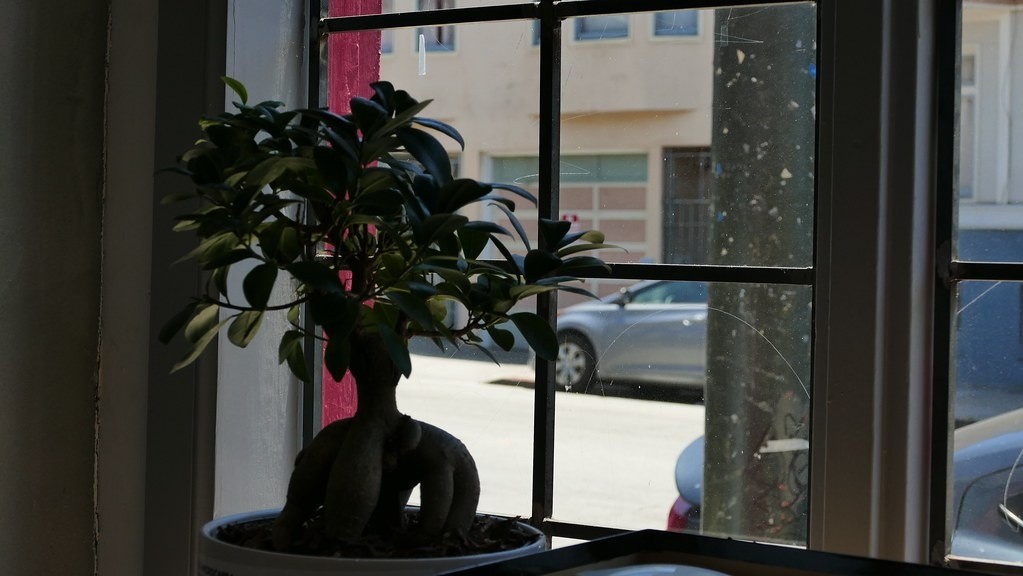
<box><xmin>149</xmin><ymin>76</ymin><xmax>631</xmax><ymax>576</ymax></box>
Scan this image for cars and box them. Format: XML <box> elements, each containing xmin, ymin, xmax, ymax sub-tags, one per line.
<box><xmin>529</xmin><ymin>272</ymin><xmax>707</xmax><ymax>394</ymax></box>
<box><xmin>662</xmin><ymin>274</ymin><xmax>1022</xmax><ymax>565</ymax></box>
